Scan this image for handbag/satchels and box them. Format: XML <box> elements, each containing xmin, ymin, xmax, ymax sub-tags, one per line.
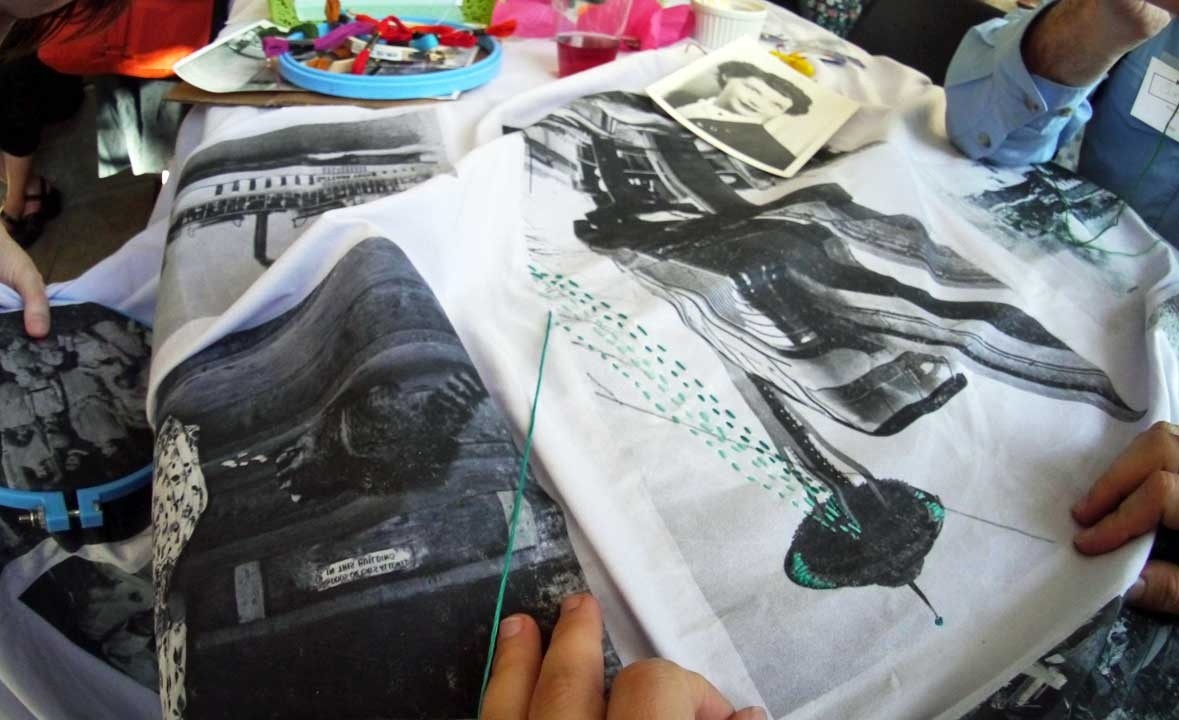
<box><xmin>36</xmin><ymin>0</ymin><xmax>215</xmax><ymax>77</ymax></box>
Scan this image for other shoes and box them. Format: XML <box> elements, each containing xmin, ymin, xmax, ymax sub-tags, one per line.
<box><xmin>0</xmin><ymin>210</ymin><xmax>20</xmax><ymax>236</ymax></box>
<box><xmin>20</xmin><ymin>174</ymin><xmax>60</xmax><ymax>248</ymax></box>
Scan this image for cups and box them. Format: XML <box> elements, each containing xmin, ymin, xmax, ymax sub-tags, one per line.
<box><xmin>554</xmin><ymin>0</ymin><xmax>633</xmax><ymax>78</ymax></box>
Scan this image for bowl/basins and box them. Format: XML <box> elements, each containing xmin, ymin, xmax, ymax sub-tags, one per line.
<box><xmin>692</xmin><ymin>0</ymin><xmax>770</xmax><ymax>51</ymax></box>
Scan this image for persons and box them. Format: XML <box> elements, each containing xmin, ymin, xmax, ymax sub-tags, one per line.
<box><xmin>946</xmin><ymin>0</ymin><xmax>1179</xmax><ymax>250</ymax></box>
<box><xmin>0</xmin><ymin>221</ymin><xmax>50</xmax><ymax>337</ymax></box>
<box><xmin>666</xmin><ymin>61</ymin><xmax>812</xmax><ymax>170</ymax></box>
<box><xmin>230</xmin><ymin>32</ymin><xmax>264</xmax><ymax>58</ymax></box>
<box><xmin>481</xmin><ymin>421</ymin><xmax>1179</xmax><ymax>720</ymax></box>
<box><xmin>0</xmin><ymin>318</ymin><xmax>153</xmax><ymax>485</ymax></box>
<box><xmin>0</xmin><ymin>0</ymin><xmax>216</xmax><ymax>250</ymax></box>
